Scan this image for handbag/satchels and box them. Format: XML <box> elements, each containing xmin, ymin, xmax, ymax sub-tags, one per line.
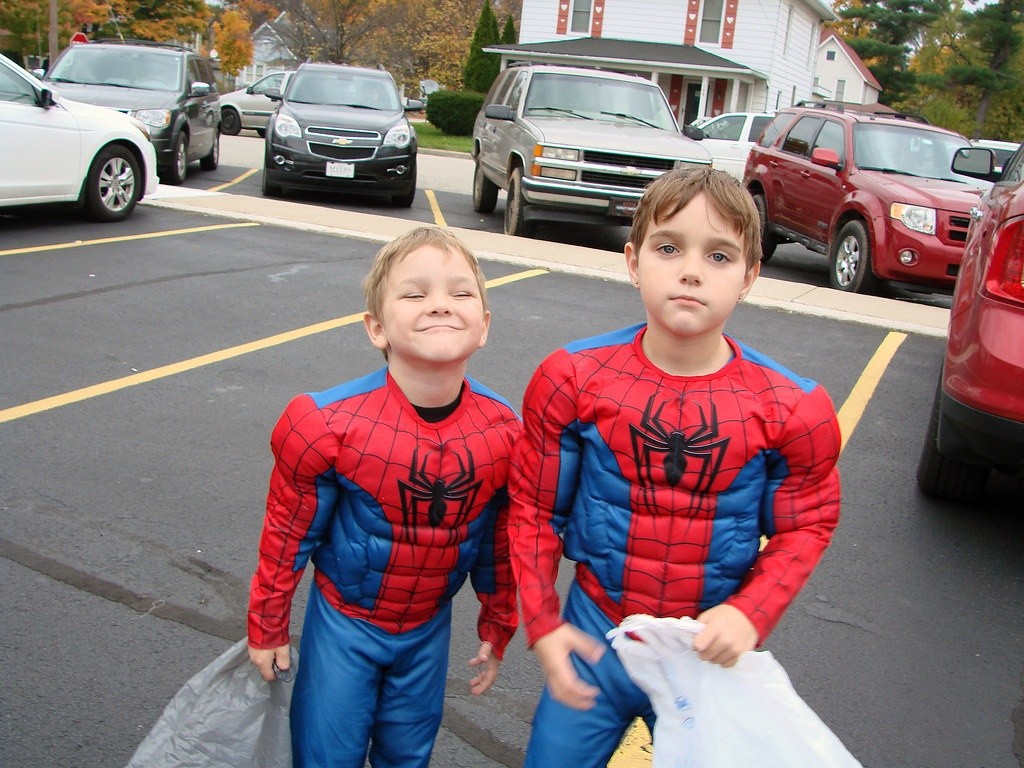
<box><xmin>604</xmin><ymin>611</ymin><xmax>861</xmax><ymax>768</ymax></box>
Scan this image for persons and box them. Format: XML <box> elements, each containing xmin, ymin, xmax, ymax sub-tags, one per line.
<box><xmin>246</xmin><ymin>226</ymin><xmax>524</xmax><ymax>768</ymax></box>
<box><xmin>508</xmin><ymin>167</ymin><xmax>842</xmax><ymax>768</ymax></box>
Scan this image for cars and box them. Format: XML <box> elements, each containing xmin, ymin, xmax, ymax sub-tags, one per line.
<box><xmin>916</xmin><ymin>144</ymin><xmax>1024</xmax><ymax>508</ymax></box>
<box><xmin>0</xmin><ymin>53</ymin><xmax>160</xmax><ymax>222</ymax></box>
<box><xmin>697</xmin><ymin>112</ymin><xmax>777</xmax><ymax>185</ymax></box>
<box><xmin>219</xmin><ymin>71</ymin><xmax>297</xmax><ymax>136</ymax></box>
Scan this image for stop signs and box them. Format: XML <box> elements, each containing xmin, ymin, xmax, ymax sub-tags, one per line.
<box><xmin>70</xmin><ymin>32</ymin><xmax>89</xmax><ymax>46</ymax></box>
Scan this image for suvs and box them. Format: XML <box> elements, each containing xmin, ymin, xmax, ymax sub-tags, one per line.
<box><xmin>470</xmin><ymin>60</ymin><xmax>714</xmax><ymax>237</ymax></box>
<box><xmin>261</xmin><ymin>56</ymin><xmax>424</xmax><ymax>209</ymax></box>
<box><xmin>744</xmin><ymin>100</ymin><xmax>995</xmax><ymax>295</ymax></box>
<box><xmin>32</xmin><ymin>40</ymin><xmax>221</xmax><ymax>186</ymax></box>
